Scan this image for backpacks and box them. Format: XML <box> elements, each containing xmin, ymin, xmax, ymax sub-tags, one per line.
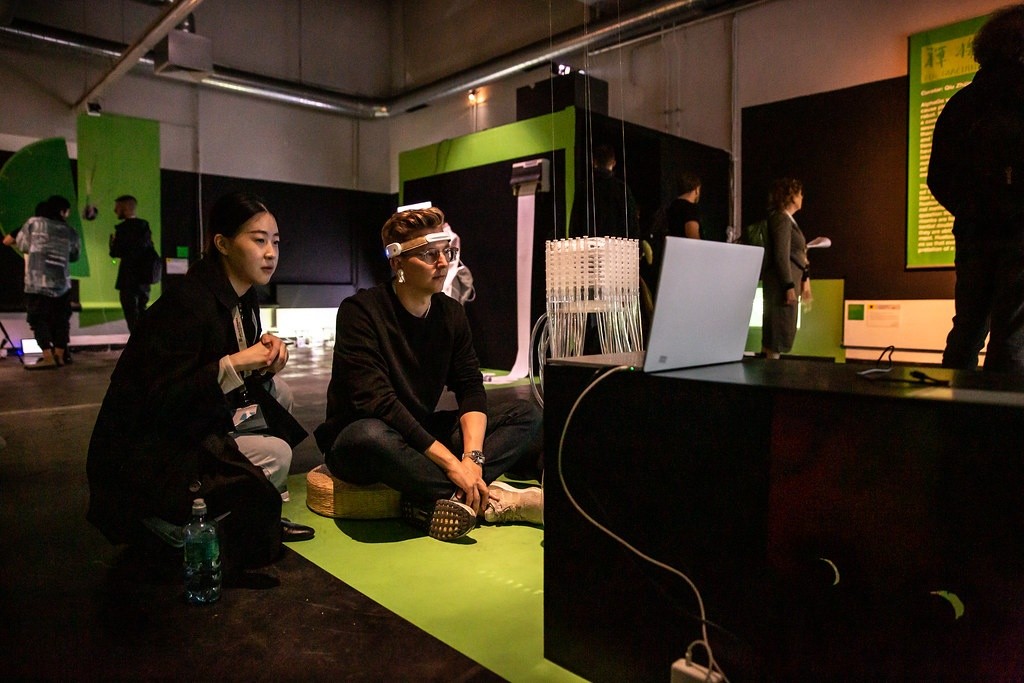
<box><xmin>741</xmin><ymin>207</ymin><xmax>780</xmax><ymax>278</ymax></box>
<box><xmin>141</xmin><ymin>239</ymin><xmax>161</xmax><ymax>283</ymax></box>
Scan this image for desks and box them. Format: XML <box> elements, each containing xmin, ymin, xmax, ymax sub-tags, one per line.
<box><xmin>543</xmin><ymin>357</ymin><xmax>1023</xmax><ymax>683</ymax></box>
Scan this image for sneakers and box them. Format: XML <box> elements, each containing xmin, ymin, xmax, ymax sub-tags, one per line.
<box><xmin>484</xmin><ymin>480</ymin><xmax>544</xmax><ymax>526</ymax></box>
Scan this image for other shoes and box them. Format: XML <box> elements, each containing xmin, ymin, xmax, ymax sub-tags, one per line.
<box><xmin>24</xmin><ymin>355</ymin><xmax>65</xmax><ymax>370</ymax></box>
<box><xmin>63</xmin><ymin>352</ymin><xmax>73</xmax><ymax>363</ymax></box>
<box><xmin>399</xmin><ymin>493</ymin><xmax>477</xmax><ymax>542</ymax></box>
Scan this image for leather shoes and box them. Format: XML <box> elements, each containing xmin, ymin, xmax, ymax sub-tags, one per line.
<box><xmin>280</xmin><ymin>516</ymin><xmax>315</xmax><ymax>542</ymax></box>
<box><xmin>229</xmin><ymin>561</ymin><xmax>282</xmax><ymax>588</ymax></box>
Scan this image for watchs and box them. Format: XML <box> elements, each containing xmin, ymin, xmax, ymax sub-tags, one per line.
<box><xmin>462</xmin><ymin>450</ymin><xmax>485</xmax><ymax>464</ymax></box>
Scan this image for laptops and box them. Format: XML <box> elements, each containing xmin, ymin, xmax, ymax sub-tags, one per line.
<box><xmin>546</xmin><ymin>236</ymin><xmax>764</xmax><ymax>372</ymax></box>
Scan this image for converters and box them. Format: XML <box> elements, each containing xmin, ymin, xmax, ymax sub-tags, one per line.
<box><xmin>671</xmin><ymin>658</ymin><xmax>723</xmax><ymax>683</ymax></box>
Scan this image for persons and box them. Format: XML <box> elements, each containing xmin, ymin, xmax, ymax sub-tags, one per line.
<box><xmin>668</xmin><ymin>171</ymin><xmax>706</xmax><ymax>241</ymax></box>
<box><xmin>574</xmin><ymin>144</ymin><xmax>641</xmax><ymax>238</ymax></box>
<box><xmin>928</xmin><ymin>5</ymin><xmax>1024</xmax><ymax>372</ymax></box>
<box><xmin>312</xmin><ymin>207</ymin><xmax>545</xmax><ymax>540</ymax></box>
<box><xmin>3</xmin><ymin>196</ymin><xmax>81</xmax><ymax>369</ymax></box>
<box><xmin>109</xmin><ymin>194</ymin><xmax>152</xmax><ymax>334</ymax></box>
<box><xmin>86</xmin><ymin>200</ymin><xmax>316</xmax><ymax>542</ymax></box>
<box><xmin>760</xmin><ymin>174</ymin><xmax>810</xmax><ymax>360</ymax></box>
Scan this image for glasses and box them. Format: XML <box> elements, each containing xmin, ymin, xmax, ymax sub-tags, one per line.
<box><xmin>404</xmin><ymin>246</ymin><xmax>459</xmax><ymax>265</ymax></box>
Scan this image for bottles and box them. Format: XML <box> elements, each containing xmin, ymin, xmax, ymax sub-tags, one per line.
<box><xmin>183</xmin><ymin>499</ymin><xmax>220</xmax><ymax>604</ymax></box>
